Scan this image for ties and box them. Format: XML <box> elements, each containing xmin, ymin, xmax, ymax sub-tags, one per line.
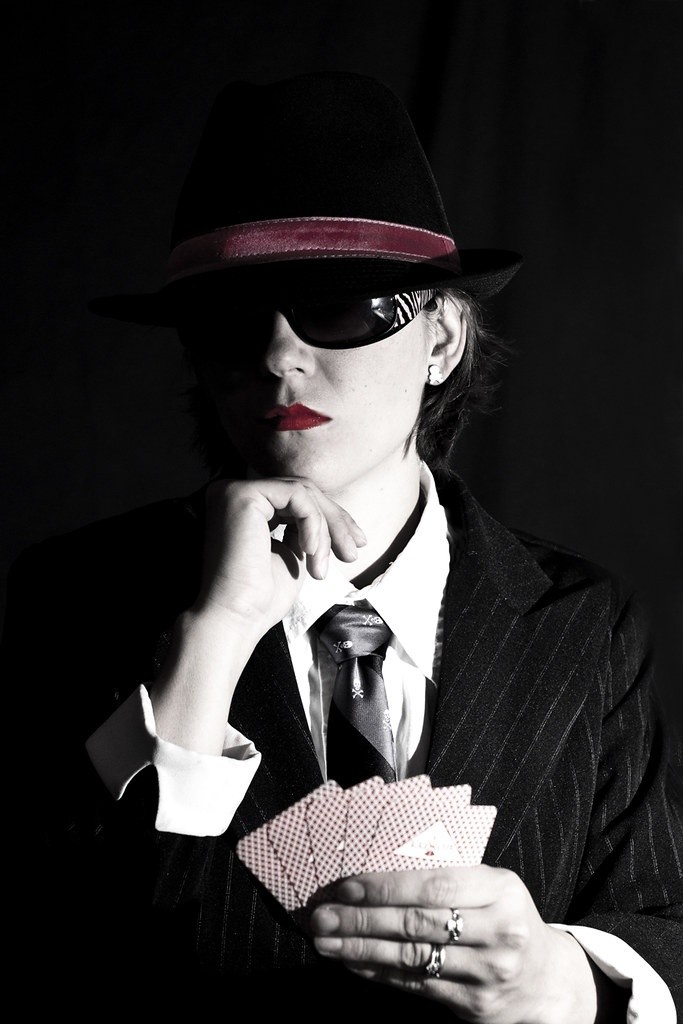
<box><xmin>308</xmin><ymin>599</ymin><xmax>397</xmax><ymax>790</ymax></box>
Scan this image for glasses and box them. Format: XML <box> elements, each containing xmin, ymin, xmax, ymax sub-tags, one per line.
<box><xmin>163</xmin><ymin>256</ymin><xmax>438</xmax><ymax>357</ymax></box>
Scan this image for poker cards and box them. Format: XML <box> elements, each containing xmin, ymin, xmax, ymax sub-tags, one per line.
<box><xmin>235</xmin><ymin>771</ymin><xmax>500</xmax><ymax>934</ymax></box>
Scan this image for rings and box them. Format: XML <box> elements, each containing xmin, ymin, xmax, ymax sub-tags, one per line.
<box><xmin>447</xmin><ymin>908</ymin><xmax>462</xmax><ymax>945</ymax></box>
<box><xmin>423</xmin><ymin>943</ymin><xmax>442</xmax><ymax>976</ymax></box>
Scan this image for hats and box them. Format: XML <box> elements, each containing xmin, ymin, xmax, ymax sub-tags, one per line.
<box><xmin>88</xmin><ymin>72</ymin><xmax>524</xmax><ymax>328</ymax></box>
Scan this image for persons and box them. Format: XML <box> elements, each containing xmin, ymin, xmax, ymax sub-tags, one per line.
<box><xmin>1</xmin><ymin>86</ymin><xmax>683</xmax><ymax>1024</ymax></box>
